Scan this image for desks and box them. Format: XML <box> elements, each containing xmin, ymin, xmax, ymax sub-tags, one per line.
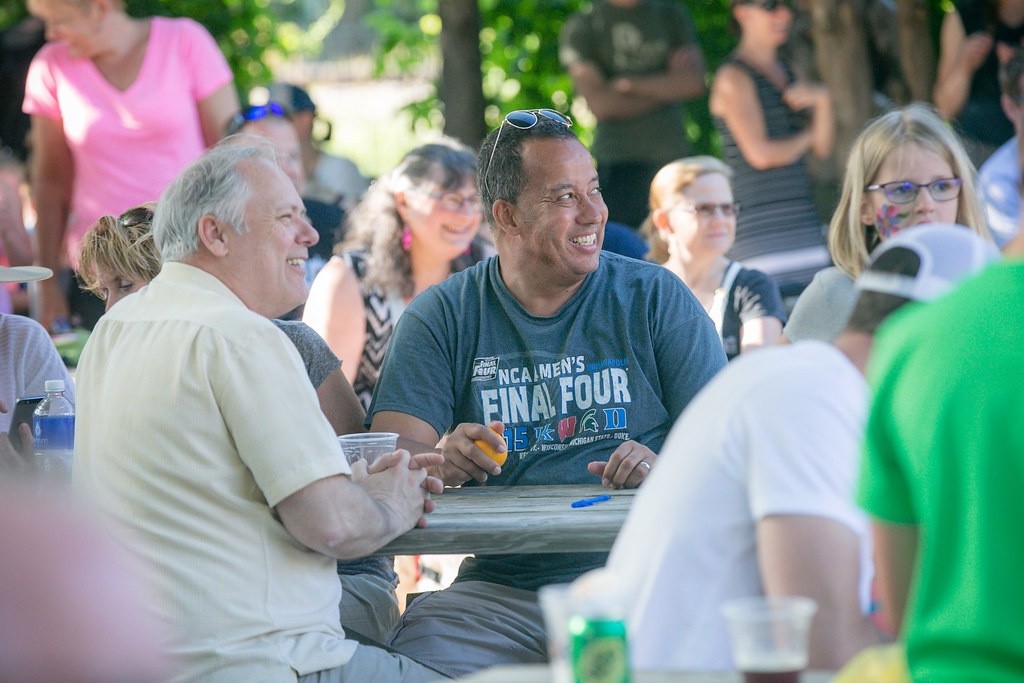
<box><xmin>350</xmin><ymin>485</ymin><xmax>637</xmax><ymax>557</ymax></box>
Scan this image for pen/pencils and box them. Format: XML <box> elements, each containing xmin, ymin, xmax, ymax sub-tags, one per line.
<box><xmin>571</xmin><ymin>495</ymin><xmax>611</xmax><ymax>508</ymax></box>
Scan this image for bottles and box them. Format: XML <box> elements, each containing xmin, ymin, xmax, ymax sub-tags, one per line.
<box><xmin>30</xmin><ymin>380</ymin><xmax>74</xmax><ymax>479</ymax></box>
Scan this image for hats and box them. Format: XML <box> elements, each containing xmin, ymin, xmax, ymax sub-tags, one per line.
<box><xmin>856</xmin><ymin>224</ymin><xmax>1001</xmax><ymax>302</ymax></box>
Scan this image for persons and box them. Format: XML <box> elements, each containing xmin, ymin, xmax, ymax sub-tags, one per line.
<box><xmin>22</xmin><ymin>0</ymin><xmax>256</xmax><ymax>333</ymax></box>
<box><xmin>0</xmin><ymin>84</ymin><xmax>498</xmax><ymax>683</ymax></box>
<box><xmin>564</xmin><ymin>0</ymin><xmax>1024</xmax><ymax>683</ymax></box>
<box><xmin>364</xmin><ymin>108</ymin><xmax>731</xmax><ymax>683</ymax></box>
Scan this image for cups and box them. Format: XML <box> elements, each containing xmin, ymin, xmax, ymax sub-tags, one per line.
<box><xmin>337</xmin><ymin>431</ymin><xmax>400</xmax><ymax>479</ymax></box>
<box><xmin>722</xmin><ymin>592</ymin><xmax>821</xmax><ymax>683</ymax></box>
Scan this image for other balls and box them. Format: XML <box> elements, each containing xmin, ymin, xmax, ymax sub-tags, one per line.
<box><xmin>473</xmin><ymin>428</ymin><xmax>508</xmax><ymax>466</ymax></box>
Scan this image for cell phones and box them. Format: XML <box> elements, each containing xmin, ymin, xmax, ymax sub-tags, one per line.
<box><xmin>8</xmin><ymin>397</ymin><xmax>45</xmax><ymax>453</ymax></box>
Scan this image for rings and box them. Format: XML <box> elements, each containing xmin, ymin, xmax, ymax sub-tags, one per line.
<box><xmin>639</xmin><ymin>462</ymin><xmax>651</xmax><ymax>470</ymax></box>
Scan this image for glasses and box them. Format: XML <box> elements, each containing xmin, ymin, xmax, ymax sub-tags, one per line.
<box><xmin>687</xmin><ymin>202</ymin><xmax>739</xmax><ymax>219</ymax></box>
<box><xmin>227</xmin><ymin>103</ymin><xmax>285</xmax><ymax>134</ymax></box>
<box><xmin>93</xmin><ymin>208</ymin><xmax>154</xmax><ymax>242</ymax></box>
<box><xmin>763</xmin><ymin>0</ymin><xmax>796</xmax><ymax>10</ymax></box>
<box><xmin>864</xmin><ymin>177</ymin><xmax>963</xmax><ymax>204</ymax></box>
<box><xmin>485</xmin><ymin>109</ymin><xmax>573</xmax><ymax>202</ymax></box>
<box><xmin>419</xmin><ymin>189</ymin><xmax>483</xmax><ymax>212</ymax></box>
